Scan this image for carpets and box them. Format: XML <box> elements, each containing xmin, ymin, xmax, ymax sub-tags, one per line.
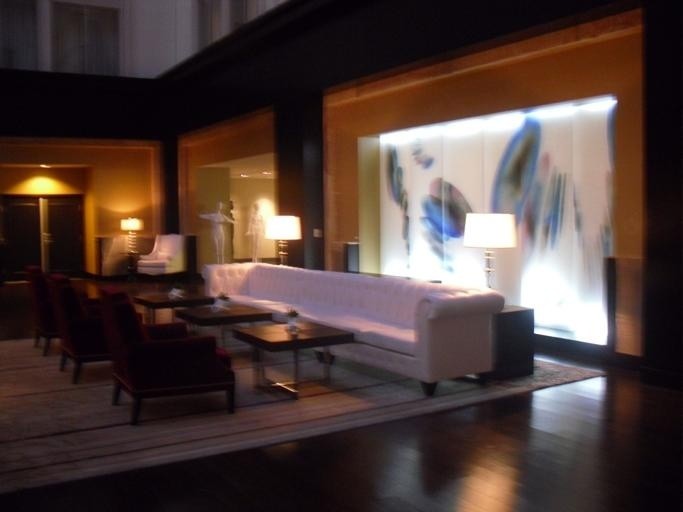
<box><xmin>1</xmin><ymin>329</ymin><xmax>610</xmax><ymax>493</ymax></box>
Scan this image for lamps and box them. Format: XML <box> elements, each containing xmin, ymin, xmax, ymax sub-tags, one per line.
<box><xmin>261</xmin><ymin>214</ymin><xmax>302</xmax><ymax>267</ymax></box>
<box><xmin>462</xmin><ymin>212</ymin><xmax>518</xmax><ymax>290</ymax></box>
<box><xmin>119</xmin><ymin>217</ymin><xmax>143</xmax><ymax>256</ymax></box>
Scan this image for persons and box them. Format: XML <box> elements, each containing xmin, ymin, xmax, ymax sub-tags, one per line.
<box><xmin>244</xmin><ymin>204</ymin><xmax>264</xmax><ymax>262</ymax></box>
<box><xmin>195</xmin><ymin>202</ymin><xmax>238</xmax><ymax>266</ymax></box>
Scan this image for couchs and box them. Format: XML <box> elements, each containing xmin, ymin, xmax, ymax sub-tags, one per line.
<box><xmin>39</xmin><ymin>273</ymin><xmax>144</xmax><ymax>386</ymax></box>
<box><xmin>104</xmin><ymin>303</ymin><xmax>238</xmax><ymax>422</ymax></box>
<box><xmin>196</xmin><ymin>259</ymin><xmax>504</xmax><ymax>399</ymax></box>
<box><xmin>19</xmin><ymin>264</ymin><xmax>81</xmax><ymax>358</ymax></box>
<box><xmin>137</xmin><ymin>231</ymin><xmax>193</xmax><ymax>285</ymax></box>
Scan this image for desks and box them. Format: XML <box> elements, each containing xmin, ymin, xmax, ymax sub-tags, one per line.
<box><xmin>121</xmin><ymin>249</ymin><xmax>143</xmax><ymax>278</ymax></box>
<box><xmin>173</xmin><ymin>300</ymin><xmax>274</xmax><ymax>369</ymax></box>
<box><xmin>71</xmin><ymin>274</ymin><xmax>143</xmax><ymax>317</ymax></box>
<box><xmin>231</xmin><ymin>320</ymin><xmax>356</xmax><ymax>399</ymax></box>
<box><xmin>466</xmin><ymin>304</ymin><xmax>537</xmax><ymax>385</ymax></box>
<box><xmin>129</xmin><ymin>290</ymin><xmax>212</xmax><ymax>330</ymax></box>
